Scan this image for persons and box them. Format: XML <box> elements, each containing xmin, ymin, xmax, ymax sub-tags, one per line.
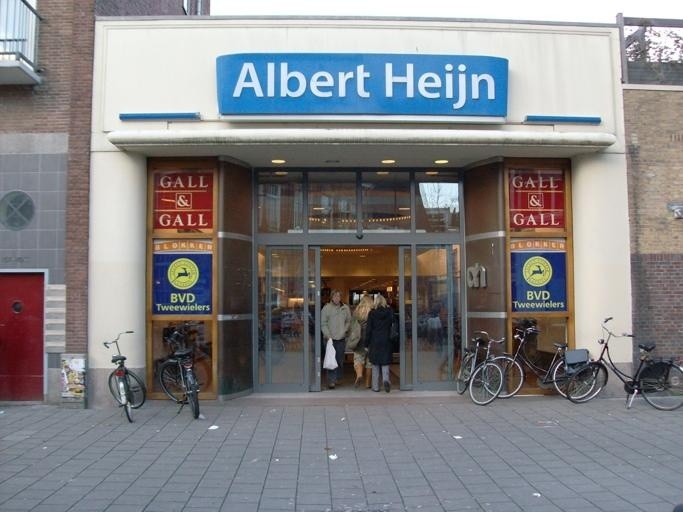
<box><xmin>352</xmin><ymin>295</ymin><xmax>373</xmax><ymax>389</ymax></box>
<box><xmin>423</xmin><ymin>311</ymin><xmax>443</xmax><ymax>353</ymax></box>
<box><xmin>363</xmin><ymin>295</ymin><xmax>394</xmax><ymax>392</ymax></box>
<box><xmin>321</xmin><ymin>288</ymin><xmax>352</xmax><ymax>389</ymax></box>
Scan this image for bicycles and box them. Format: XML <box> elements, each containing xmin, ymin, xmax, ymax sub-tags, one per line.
<box><xmin>159</xmin><ymin>322</ymin><xmax>201</xmax><ymax>419</ymax></box>
<box><xmin>439</xmin><ymin>332</ymin><xmax>462</xmax><ymax>380</ymax></box>
<box><xmin>457</xmin><ymin>331</ymin><xmax>506</xmax><ymax>405</ymax></box>
<box><xmin>567</xmin><ymin>317</ymin><xmax>683</xmax><ymax>410</ymax></box>
<box><xmin>104</xmin><ymin>331</ymin><xmax>146</xmax><ymax>423</ymax></box>
<box><xmin>483</xmin><ymin>326</ymin><xmax>596</xmax><ymax>400</ymax></box>
<box><xmin>259</xmin><ymin>328</ymin><xmax>285</xmax><ymax>364</ymax></box>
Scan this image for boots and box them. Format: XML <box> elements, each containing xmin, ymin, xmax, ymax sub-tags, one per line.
<box><xmin>355</xmin><ymin>365</ymin><xmax>363</xmax><ymax>384</ymax></box>
<box><xmin>365</xmin><ymin>368</ymin><xmax>372</xmax><ymax>388</ymax></box>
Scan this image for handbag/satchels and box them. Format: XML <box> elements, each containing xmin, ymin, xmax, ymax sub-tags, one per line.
<box><xmin>348</xmin><ymin>320</ymin><xmax>361</xmax><ymax>349</ymax></box>
<box><xmin>390</xmin><ymin>323</ymin><xmax>399</xmax><ymax>341</ymax></box>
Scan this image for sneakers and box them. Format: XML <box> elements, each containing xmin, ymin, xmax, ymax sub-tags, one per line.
<box><xmin>384</xmin><ymin>381</ymin><xmax>390</xmax><ymax>393</ymax></box>
<box><xmin>329</xmin><ymin>379</ymin><xmax>342</xmax><ymax>388</ymax></box>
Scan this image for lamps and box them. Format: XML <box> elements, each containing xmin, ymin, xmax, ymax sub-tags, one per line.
<box><xmin>309</xmin><ymin>188</ymin><xmax>410</xmax><ymax>222</ymax></box>
